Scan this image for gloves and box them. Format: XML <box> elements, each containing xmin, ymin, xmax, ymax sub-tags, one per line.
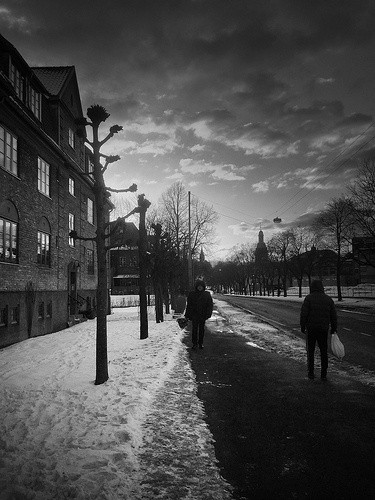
<box><xmin>330</xmin><ymin>329</ymin><xmax>337</xmax><ymax>334</ymax></box>
<box><xmin>301</xmin><ymin>327</ymin><xmax>306</xmax><ymax>333</ymax></box>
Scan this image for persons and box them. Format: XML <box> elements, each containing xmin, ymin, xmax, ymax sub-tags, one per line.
<box><xmin>184</xmin><ymin>281</ymin><xmax>212</xmax><ymax>348</ymax></box>
<box><xmin>299</xmin><ymin>280</ymin><xmax>338</xmax><ymax>382</ymax></box>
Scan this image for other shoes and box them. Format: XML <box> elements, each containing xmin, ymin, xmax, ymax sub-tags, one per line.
<box><xmin>309</xmin><ymin>373</ymin><xmax>314</xmax><ymax>379</ymax></box>
<box><xmin>322</xmin><ymin>377</ymin><xmax>327</xmax><ymax>381</ymax></box>
<box><xmin>199</xmin><ymin>343</ymin><xmax>204</xmax><ymax>349</ymax></box>
<box><xmin>192</xmin><ymin>342</ymin><xmax>198</xmax><ymax>349</ymax></box>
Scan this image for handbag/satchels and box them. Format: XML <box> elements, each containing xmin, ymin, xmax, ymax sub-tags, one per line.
<box><xmin>331</xmin><ymin>332</ymin><xmax>345</xmax><ymax>358</ymax></box>
